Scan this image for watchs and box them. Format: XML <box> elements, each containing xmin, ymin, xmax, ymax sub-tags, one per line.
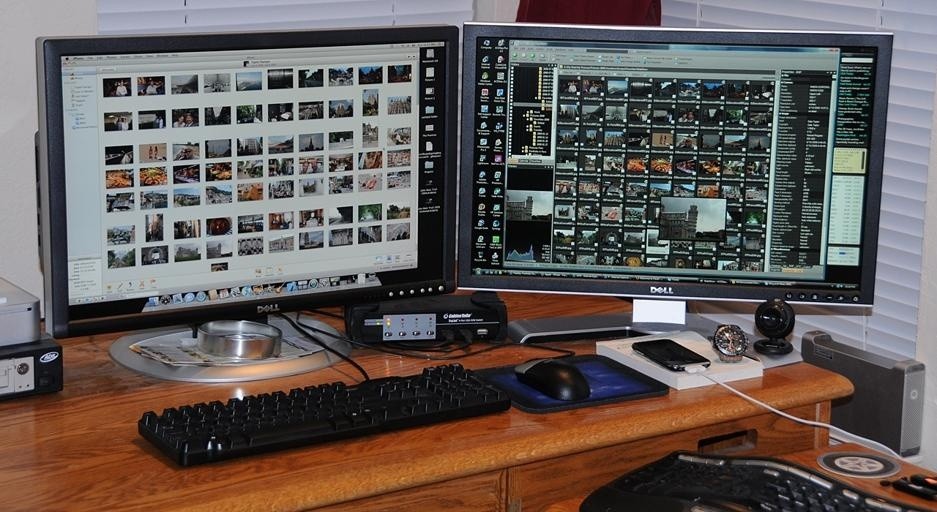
<box><xmin>712</xmin><ymin>323</ymin><xmax>749</xmax><ymax>362</ymax></box>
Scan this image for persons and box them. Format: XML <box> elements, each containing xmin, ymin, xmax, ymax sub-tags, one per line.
<box><xmin>554</xmin><ymin>183</ymin><xmax>575</xmax><ymax>198</ymax></box>
<box><xmin>677</xmin><ymin>112</ymin><xmax>698</xmax><ymax>127</ymax></box>
<box><xmin>603</xmin><ymin>210</ymin><xmax>619</xmax><ymax>221</ymax></box>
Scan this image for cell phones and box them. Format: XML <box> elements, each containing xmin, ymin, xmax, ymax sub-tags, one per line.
<box><xmin>633</xmin><ymin>339</ymin><xmax>711</xmax><ymax>373</ymax></box>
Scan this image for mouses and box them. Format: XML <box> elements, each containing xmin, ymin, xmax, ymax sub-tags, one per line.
<box><xmin>513</xmin><ymin>357</ymin><xmax>592</xmax><ymax>403</ymax></box>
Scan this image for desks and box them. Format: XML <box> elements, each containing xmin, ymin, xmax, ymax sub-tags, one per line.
<box><xmin>546</xmin><ymin>441</ymin><xmax>937</xmax><ymax>511</ymax></box>
<box><xmin>1</xmin><ymin>289</ymin><xmax>856</xmax><ymax>512</ymax></box>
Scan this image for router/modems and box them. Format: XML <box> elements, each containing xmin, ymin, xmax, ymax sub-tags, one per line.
<box><xmin>802</xmin><ymin>329</ymin><xmax>925</xmax><ymax>457</ymax></box>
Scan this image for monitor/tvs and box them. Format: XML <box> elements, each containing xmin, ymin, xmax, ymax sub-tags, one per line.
<box><xmin>456</xmin><ymin>19</ymin><xmax>896</xmax><ymax>367</ymax></box>
<box><xmin>35</xmin><ymin>23</ymin><xmax>459</xmax><ymax>383</ymax></box>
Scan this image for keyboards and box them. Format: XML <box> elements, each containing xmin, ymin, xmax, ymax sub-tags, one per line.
<box><xmin>137</xmin><ymin>364</ymin><xmax>512</xmax><ymax>469</ymax></box>
<box><xmin>579</xmin><ymin>450</ymin><xmax>936</xmax><ymax>512</ymax></box>
